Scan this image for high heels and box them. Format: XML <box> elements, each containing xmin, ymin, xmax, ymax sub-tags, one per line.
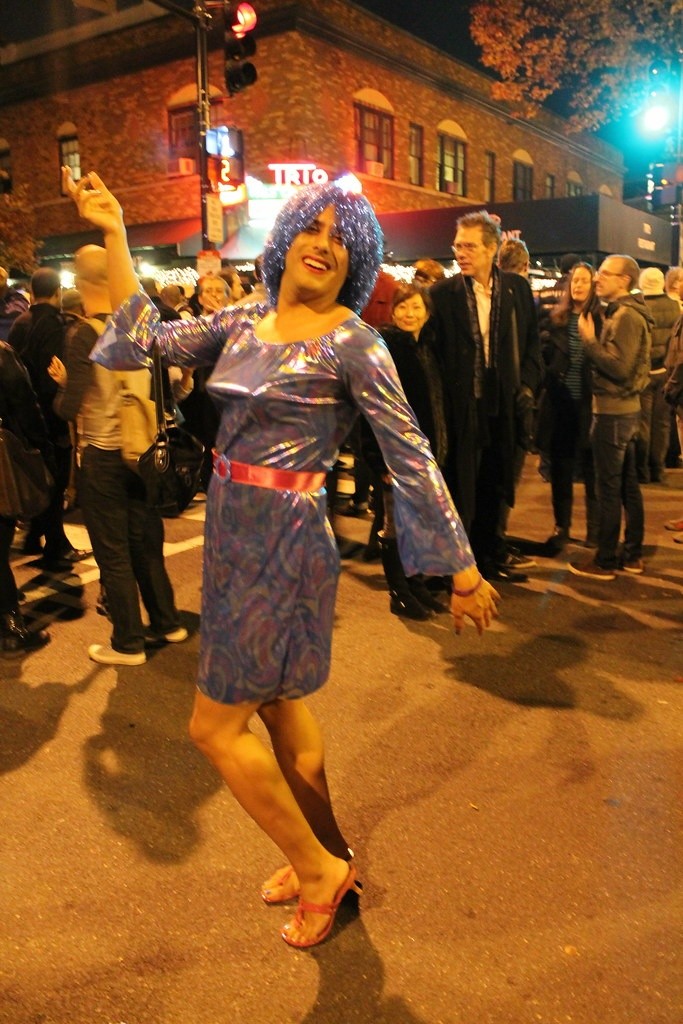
<box><xmin>259</xmin><ymin>848</ymin><xmax>355</xmax><ymax>903</ymax></box>
<box><xmin>283</xmin><ymin>862</ymin><xmax>363</xmax><ymax>948</ymax></box>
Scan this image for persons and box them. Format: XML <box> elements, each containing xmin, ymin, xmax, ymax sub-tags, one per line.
<box><xmin>427</xmin><ymin>212</ymin><xmax>543</xmax><ymax>584</ymax></box>
<box><xmin>144</xmin><ymin>257</ymin><xmax>268</xmax><ymax>497</ymax></box>
<box><xmin>413</xmin><ymin>258</ymin><xmax>445</xmax><ymax>288</ymax></box>
<box><xmin>46</xmin><ymin>245</ymin><xmax>188</xmax><ymax>667</ymax></box>
<box><xmin>0</xmin><ymin>267</ymin><xmax>95</xmax><ymax>652</ymax></box>
<box><xmin>569</xmin><ymin>255</ymin><xmax>654</xmax><ymax>580</ymax></box>
<box><xmin>498</xmin><ymin>238</ymin><xmax>530</xmax><ymax>278</ymax></box>
<box><xmin>540</xmin><ymin>263</ymin><xmax>603</xmax><ymax>548</ymax></box>
<box><xmin>638</xmin><ymin>263</ymin><xmax>683</xmax><ymax>483</ymax></box>
<box><xmin>62</xmin><ymin>164</ymin><xmax>502</xmax><ymax>948</ymax></box>
<box><xmin>335</xmin><ymin>271</ymin><xmax>400</xmax><ymax>516</ymax></box>
<box><xmin>363</xmin><ymin>286</ymin><xmax>449</xmax><ymax>620</ymax></box>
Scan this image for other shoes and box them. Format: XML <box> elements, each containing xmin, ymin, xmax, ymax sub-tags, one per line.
<box><xmin>664</xmin><ymin>519</ymin><xmax>683</xmax><ymax>530</ymax></box>
<box><xmin>44</xmin><ymin>549</ymin><xmax>87</xmax><ymax>561</ymax></box>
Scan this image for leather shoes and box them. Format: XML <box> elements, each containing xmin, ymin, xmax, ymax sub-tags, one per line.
<box><xmin>486</xmin><ymin>566</ymin><xmax>529</xmax><ymax>584</ymax></box>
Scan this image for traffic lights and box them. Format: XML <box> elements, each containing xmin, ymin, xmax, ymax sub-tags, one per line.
<box><xmin>223</xmin><ymin>0</ymin><xmax>259</xmax><ymax>93</ymax></box>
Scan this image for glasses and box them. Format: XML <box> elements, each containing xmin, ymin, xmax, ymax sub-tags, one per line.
<box><xmin>451</xmin><ymin>242</ymin><xmax>481</xmax><ymax>252</ymax></box>
<box><xmin>597</xmin><ymin>270</ymin><xmax>624</xmax><ymax>280</ymax></box>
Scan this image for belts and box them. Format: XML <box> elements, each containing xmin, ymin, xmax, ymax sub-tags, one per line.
<box><xmin>212</xmin><ymin>448</ymin><xmax>326</xmax><ymax>492</ymax></box>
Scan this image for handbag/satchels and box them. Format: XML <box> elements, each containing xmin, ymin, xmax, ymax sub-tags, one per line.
<box><xmin>136</xmin><ymin>339</ymin><xmax>206</xmax><ymax>519</ymax></box>
<box><xmin>120</xmin><ymin>390</ymin><xmax>177</xmax><ymax>473</ymax></box>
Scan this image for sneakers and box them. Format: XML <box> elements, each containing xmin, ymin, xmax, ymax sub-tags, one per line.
<box><xmin>568</xmin><ymin>558</ymin><xmax>616</xmax><ymax>580</ymax></box>
<box><xmin>548</xmin><ymin>526</ymin><xmax>569</xmax><ymax>548</ymax></box>
<box><xmin>585</xmin><ymin>533</ymin><xmax>600</xmax><ymax>547</ymax></box>
<box><xmin>89</xmin><ymin>645</ymin><xmax>147</xmax><ymax>666</ymax></box>
<box><xmin>617</xmin><ymin>555</ymin><xmax>645</xmax><ymax>574</ymax></box>
<box><xmin>497</xmin><ymin>549</ymin><xmax>535</xmax><ymax>569</ymax></box>
<box><xmin>145</xmin><ymin>628</ymin><xmax>188</xmax><ymax>644</ymax></box>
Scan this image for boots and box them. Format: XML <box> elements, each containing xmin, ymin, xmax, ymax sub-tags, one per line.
<box><xmin>2</xmin><ymin>609</ymin><xmax>50</xmax><ymax>658</ymax></box>
<box><xmin>382</xmin><ymin>537</ymin><xmax>435</xmax><ymax>620</ymax></box>
<box><xmin>413</xmin><ymin>580</ymin><xmax>447</xmax><ymax>614</ymax></box>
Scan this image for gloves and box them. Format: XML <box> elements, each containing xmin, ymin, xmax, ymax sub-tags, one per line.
<box><xmin>516</xmin><ymin>386</ymin><xmax>535</xmax><ymax>415</ymax></box>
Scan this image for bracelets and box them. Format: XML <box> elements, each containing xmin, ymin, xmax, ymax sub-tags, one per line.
<box><xmin>451</xmin><ymin>573</ymin><xmax>482</xmax><ymax>596</ymax></box>
